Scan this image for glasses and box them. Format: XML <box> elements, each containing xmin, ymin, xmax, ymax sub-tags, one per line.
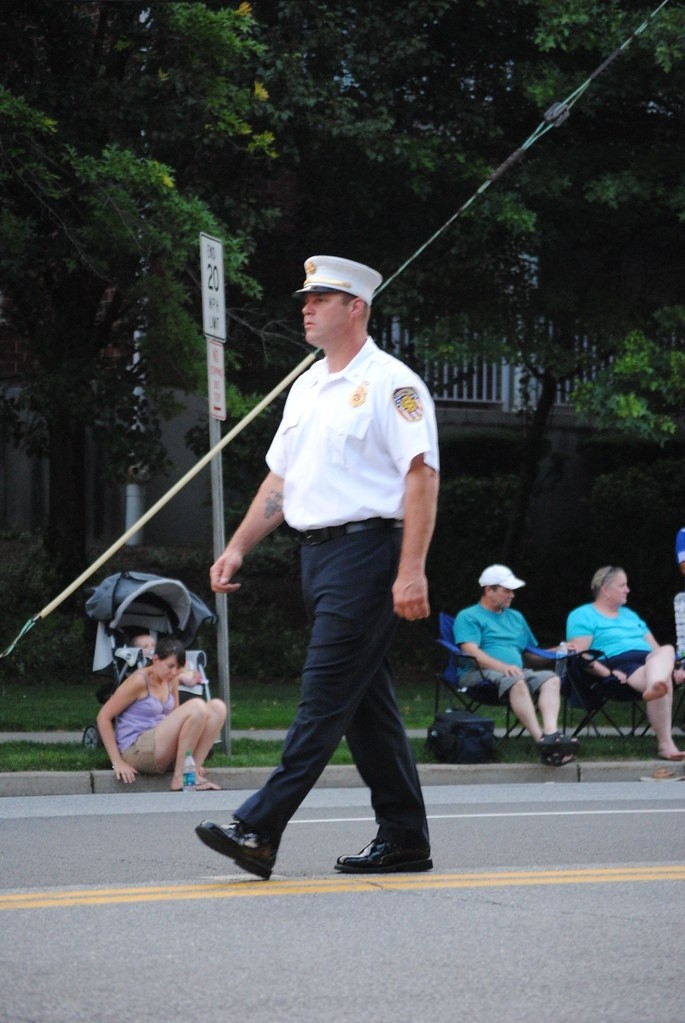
<box><xmin>600</xmin><ymin>567</ymin><xmax>615</xmax><ymax>592</ymax></box>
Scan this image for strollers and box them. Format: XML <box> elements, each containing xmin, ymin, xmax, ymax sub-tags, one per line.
<box><xmin>81</xmin><ymin>570</ymin><xmax>219</xmax><ymax>750</ymax></box>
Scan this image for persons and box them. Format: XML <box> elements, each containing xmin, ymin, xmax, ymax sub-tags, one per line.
<box><xmin>97</xmin><ymin>638</ymin><xmax>227</xmax><ymax>791</ymax></box>
<box><xmin>133</xmin><ymin>635</ymin><xmax>203</xmax><ymax>686</ymax></box>
<box><xmin>677</xmin><ymin>528</ymin><xmax>685</xmax><ymax>576</ymax></box>
<box><xmin>452</xmin><ymin>564</ymin><xmax>579</xmax><ymax>767</ymax></box>
<box><xmin>566</xmin><ymin>566</ymin><xmax>685</xmax><ymax>760</ymax></box>
<box><xmin>194</xmin><ymin>256</ymin><xmax>440</xmax><ymax>879</ymax></box>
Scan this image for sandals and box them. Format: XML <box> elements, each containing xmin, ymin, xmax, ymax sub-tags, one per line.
<box><xmin>536</xmin><ymin>731</ymin><xmax>579</xmax><ymax>754</ymax></box>
<box><xmin>541</xmin><ymin>751</ymin><xmax>574</xmax><ymax>766</ymax></box>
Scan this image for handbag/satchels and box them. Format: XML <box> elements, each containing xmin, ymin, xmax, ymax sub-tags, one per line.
<box><xmin>424</xmin><ymin>709</ymin><xmax>501</xmax><ymax>764</ymax></box>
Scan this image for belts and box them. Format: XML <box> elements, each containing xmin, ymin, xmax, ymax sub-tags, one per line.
<box><xmin>301</xmin><ymin>517</ymin><xmax>404</xmax><ymax>547</ymax></box>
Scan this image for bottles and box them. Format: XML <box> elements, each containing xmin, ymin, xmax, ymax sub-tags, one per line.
<box><xmin>182</xmin><ymin>751</ymin><xmax>196</xmax><ymax>792</ymax></box>
<box><xmin>554</xmin><ymin>642</ymin><xmax>568</xmax><ymax>681</ymax></box>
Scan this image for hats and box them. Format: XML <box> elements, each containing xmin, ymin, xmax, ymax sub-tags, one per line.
<box><xmin>294</xmin><ymin>256</ymin><xmax>382</xmax><ymax>306</ymax></box>
<box><xmin>480</xmin><ymin>564</ymin><xmax>525</xmax><ymax>590</ymax></box>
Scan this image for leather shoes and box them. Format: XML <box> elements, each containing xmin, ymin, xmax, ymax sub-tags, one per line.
<box><xmin>195</xmin><ymin>815</ymin><xmax>279</xmax><ymax>880</ymax></box>
<box><xmin>334</xmin><ymin>838</ymin><xmax>433</xmax><ymax>873</ymax></box>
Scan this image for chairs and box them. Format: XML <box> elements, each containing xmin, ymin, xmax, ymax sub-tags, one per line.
<box><xmin>567</xmin><ymin>651</ymin><xmax>685</xmax><ymax>743</ymax></box>
<box><xmin>432</xmin><ymin>613</ymin><xmax>604</xmax><ymax>760</ymax></box>
